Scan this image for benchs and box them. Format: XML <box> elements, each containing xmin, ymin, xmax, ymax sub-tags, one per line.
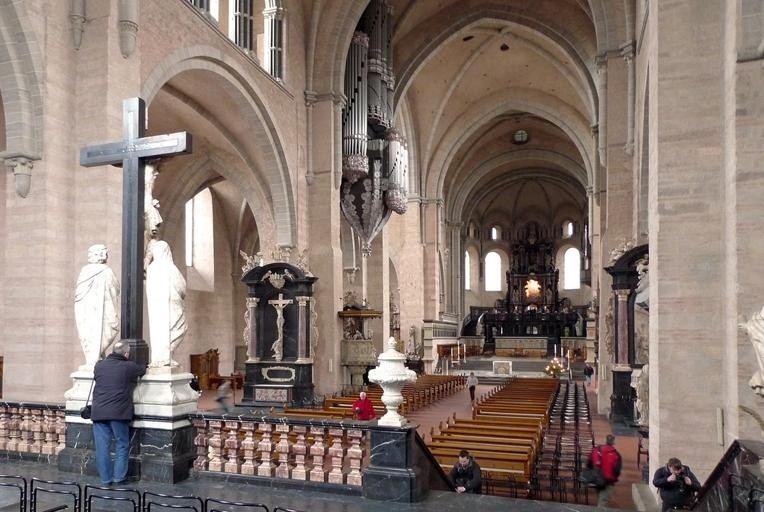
<box><xmin>422</xmin><ymin>376</ymin><xmax>561</xmax><ymax>498</ymax></box>
<box><xmin>269</xmin><ymin>384</ymin><xmax>387</xmax><ymax>418</ymax></box>
<box><xmin>400</xmin><ymin>372</ymin><xmax>469</xmax><ymax>416</ymax></box>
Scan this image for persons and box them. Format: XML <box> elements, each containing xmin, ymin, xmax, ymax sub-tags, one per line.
<box><xmin>586</xmin><ymin>434</ymin><xmax>622</xmax><ymax>507</ymax></box>
<box><xmin>464</xmin><ymin>371</ymin><xmax>478</xmax><ymax>400</ymax></box>
<box><xmin>352</xmin><ymin>391</ymin><xmax>376</xmax><ymax>421</ymax></box>
<box><xmin>212</xmin><ymin>378</ymin><xmax>236</xmax><ymax>415</ymax></box>
<box><xmin>89</xmin><ymin>342</ymin><xmax>146</xmax><ymax>488</ymax></box>
<box><xmin>142</xmin><ymin>198</ymin><xmax>190</xmax><ymax>372</ymax></box>
<box><xmin>584</xmin><ymin>362</ymin><xmax>594</xmax><ymax>385</ymax></box>
<box><xmin>271</xmin><ymin>300</ymin><xmax>290</xmax><ymax>338</ymax></box>
<box><xmin>652</xmin><ymin>456</ymin><xmax>702</xmax><ymax>512</ymax></box>
<box><xmin>446</xmin><ymin>449</ymin><xmax>481</xmax><ymax>494</ymax></box>
<box><xmin>73</xmin><ymin>244</ymin><xmax>121</xmax><ymax>366</ymax></box>
<box><xmin>186</xmin><ymin>373</ymin><xmax>203</xmax><ymax>411</ymax></box>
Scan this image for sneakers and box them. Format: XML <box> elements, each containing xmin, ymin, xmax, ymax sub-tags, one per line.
<box><xmin>103</xmin><ymin>477</ymin><xmax>132</xmax><ymax>487</ymax></box>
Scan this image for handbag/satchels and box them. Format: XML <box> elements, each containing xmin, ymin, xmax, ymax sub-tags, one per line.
<box><xmin>80</xmin><ymin>405</ymin><xmax>91</xmax><ymax>419</ymax></box>
<box><xmin>577</xmin><ymin>468</ymin><xmax>607</xmax><ymax>490</ymax></box>
